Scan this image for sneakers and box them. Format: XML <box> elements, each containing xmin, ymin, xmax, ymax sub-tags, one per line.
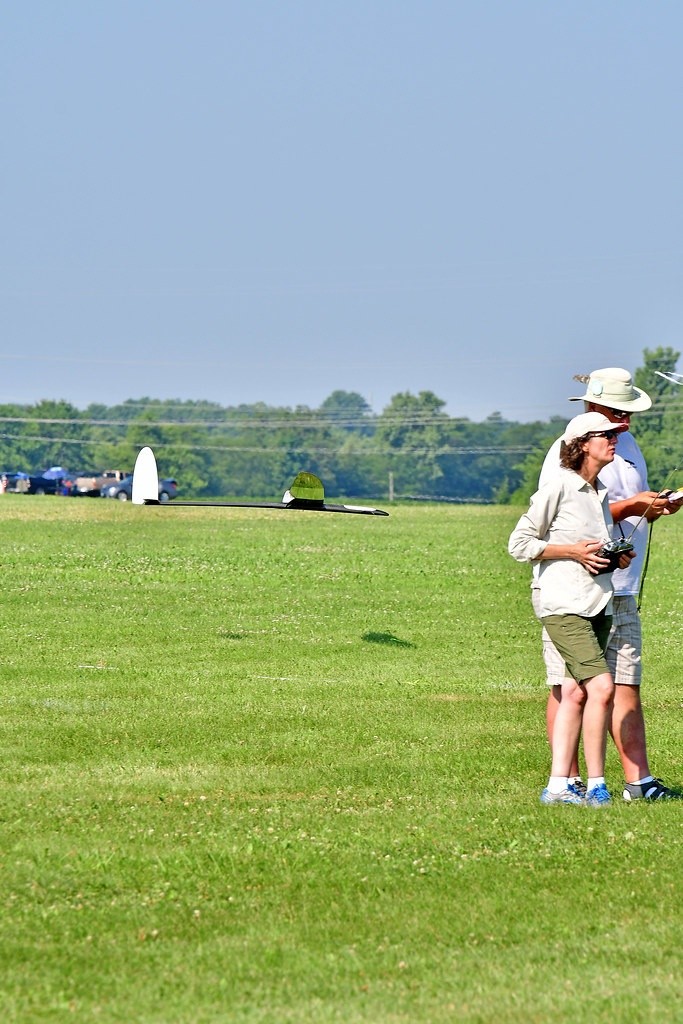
<box><xmin>584</xmin><ymin>783</ymin><xmax>611</xmax><ymax>807</ymax></box>
<box><xmin>539</xmin><ymin>783</ymin><xmax>585</xmax><ymax>805</ymax></box>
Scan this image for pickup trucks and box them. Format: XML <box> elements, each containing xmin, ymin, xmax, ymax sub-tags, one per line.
<box><xmin>76</xmin><ymin>470</ymin><xmax>134</xmax><ymax>498</ymax></box>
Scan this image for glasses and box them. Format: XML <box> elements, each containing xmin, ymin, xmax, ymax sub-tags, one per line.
<box><xmin>585</xmin><ymin>428</ymin><xmax>619</xmax><ymax>440</ymax></box>
<box><xmin>605</xmin><ymin>407</ymin><xmax>634</xmax><ymax>419</ymax></box>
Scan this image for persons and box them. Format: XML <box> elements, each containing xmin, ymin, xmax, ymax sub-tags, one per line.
<box><xmin>538</xmin><ymin>367</ymin><xmax>683</xmax><ymax>802</ymax></box>
<box><xmin>509</xmin><ymin>411</ymin><xmax>636</xmax><ymax>806</ymax></box>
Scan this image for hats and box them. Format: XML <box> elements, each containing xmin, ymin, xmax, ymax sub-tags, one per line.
<box><xmin>568</xmin><ymin>368</ymin><xmax>652</xmax><ymax>412</ymax></box>
<box><xmin>564</xmin><ymin>412</ymin><xmax>629</xmax><ymax>447</ymax></box>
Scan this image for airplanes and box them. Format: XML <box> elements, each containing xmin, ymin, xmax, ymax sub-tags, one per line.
<box><xmin>131</xmin><ymin>446</ymin><xmax>390</xmax><ymax>517</ymax></box>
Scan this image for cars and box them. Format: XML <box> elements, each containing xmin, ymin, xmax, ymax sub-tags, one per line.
<box><xmin>100</xmin><ymin>478</ymin><xmax>178</xmax><ymax>502</ymax></box>
<box><xmin>0</xmin><ymin>467</ymin><xmax>102</xmax><ymax>499</ymax></box>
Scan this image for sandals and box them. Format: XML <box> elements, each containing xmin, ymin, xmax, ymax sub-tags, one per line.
<box><xmin>571</xmin><ymin>781</ymin><xmax>587</xmax><ymax>799</ymax></box>
<box><xmin>622</xmin><ymin>779</ymin><xmax>683</xmax><ymax>802</ymax></box>
